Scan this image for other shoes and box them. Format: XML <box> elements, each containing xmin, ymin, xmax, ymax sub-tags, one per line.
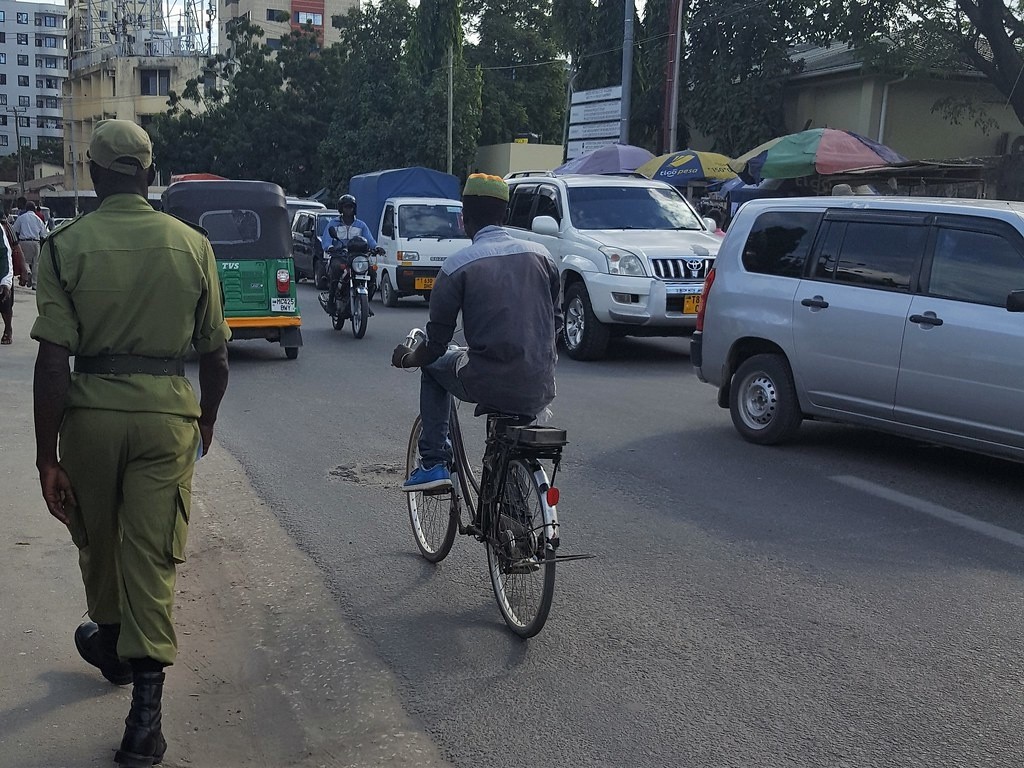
<box><xmin>32</xmin><ymin>288</ymin><xmax>35</xmax><ymax>290</ymax></box>
<box><xmin>367</xmin><ymin>306</ymin><xmax>374</xmax><ymax>316</ymax></box>
<box><xmin>27</xmin><ymin>272</ymin><xmax>32</xmax><ymax>287</ymax></box>
<box><xmin>327</xmin><ymin>299</ymin><xmax>334</xmax><ymax>309</ymax></box>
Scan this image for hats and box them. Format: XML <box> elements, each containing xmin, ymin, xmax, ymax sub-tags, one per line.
<box><xmin>832</xmin><ymin>184</ymin><xmax>856</xmax><ymax>196</ymax></box>
<box><xmin>88</xmin><ymin>118</ymin><xmax>152</xmax><ymax>175</ymax></box>
<box><xmin>463</xmin><ymin>173</ymin><xmax>509</xmax><ymax>202</ymax></box>
<box><xmin>854</xmin><ymin>184</ymin><xmax>875</xmax><ymax>196</ymax></box>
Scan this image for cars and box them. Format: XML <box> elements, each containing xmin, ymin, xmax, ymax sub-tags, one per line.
<box><xmin>290</xmin><ymin>209</ymin><xmax>346</xmax><ymax>291</ymax></box>
<box><xmin>286</xmin><ymin>197</ymin><xmax>328</xmax><ymax>227</ymax></box>
<box><xmin>8</xmin><ymin>207</ymin><xmax>73</xmax><ymax>234</ymax></box>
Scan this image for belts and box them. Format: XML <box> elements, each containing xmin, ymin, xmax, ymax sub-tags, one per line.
<box><xmin>21</xmin><ymin>239</ymin><xmax>40</xmax><ymax>241</ymax></box>
<box><xmin>74</xmin><ymin>354</ymin><xmax>186</xmax><ymax>378</ymax></box>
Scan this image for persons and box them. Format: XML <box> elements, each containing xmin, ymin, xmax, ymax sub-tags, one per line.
<box><xmin>2</xmin><ymin>210</ymin><xmax>15</xmax><ymax>225</ymax></box>
<box><xmin>12</xmin><ymin>200</ymin><xmax>48</xmax><ymax>289</ymax></box>
<box><xmin>35</xmin><ymin>206</ymin><xmax>45</xmax><ymax>222</ymax></box>
<box><xmin>392</xmin><ymin>174</ymin><xmax>558</xmax><ymax>526</ymax></box>
<box><xmin>690</xmin><ymin>191</ymin><xmax>727</xmax><ymax>239</ymax></box>
<box><xmin>322</xmin><ymin>193</ymin><xmax>386</xmax><ymax>317</ymax></box>
<box><xmin>0</xmin><ymin>222</ymin><xmax>15</xmax><ymax>344</ymax></box>
<box><xmin>29</xmin><ymin>118</ymin><xmax>233</xmax><ymax>768</ymax></box>
<box><xmin>16</xmin><ymin>197</ymin><xmax>28</xmax><ymax>216</ymax></box>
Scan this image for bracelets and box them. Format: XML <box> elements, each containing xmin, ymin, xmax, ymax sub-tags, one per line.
<box><xmin>401</xmin><ymin>352</ymin><xmax>419</xmax><ymax>373</ymax></box>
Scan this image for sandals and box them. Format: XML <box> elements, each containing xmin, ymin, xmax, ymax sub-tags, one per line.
<box><xmin>1</xmin><ymin>327</ymin><xmax>12</xmax><ymax>345</ymax></box>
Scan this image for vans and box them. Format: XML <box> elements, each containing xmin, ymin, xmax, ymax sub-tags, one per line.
<box><xmin>689</xmin><ymin>196</ymin><xmax>1024</xmax><ymax>464</ymax></box>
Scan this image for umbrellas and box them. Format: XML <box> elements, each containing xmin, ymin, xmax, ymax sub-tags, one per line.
<box><xmin>634</xmin><ymin>141</ymin><xmax>737</xmax><ymax>206</ymax></box>
<box><xmin>553</xmin><ymin>139</ymin><xmax>660</xmax><ymax>175</ymax></box>
<box><xmin>727</xmin><ymin>123</ymin><xmax>909</xmax><ymax>194</ymax></box>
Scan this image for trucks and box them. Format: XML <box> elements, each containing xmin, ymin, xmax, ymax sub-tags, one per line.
<box><xmin>352</xmin><ymin>166</ymin><xmax>474</xmax><ymax>307</ymax></box>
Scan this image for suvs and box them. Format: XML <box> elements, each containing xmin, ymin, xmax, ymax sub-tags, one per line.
<box><xmin>503</xmin><ymin>170</ymin><xmax>726</xmax><ymax>363</ymax></box>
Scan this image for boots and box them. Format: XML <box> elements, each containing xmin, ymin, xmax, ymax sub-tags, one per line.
<box><xmin>74</xmin><ymin>621</ymin><xmax>134</xmax><ymax>684</ymax></box>
<box><xmin>114</xmin><ymin>672</ymin><xmax>168</xmax><ymax>768</ymax></box>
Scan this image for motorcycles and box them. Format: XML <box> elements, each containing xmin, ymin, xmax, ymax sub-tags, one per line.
<box><xmin>159</xmin><ymin>179</ymin><xmax>303</xmax><ymax>360</ymax></box>
<box><xmin>318</xmin><ymin>226</ymin><xmax>388</xmax><ymax>338</ymax></box>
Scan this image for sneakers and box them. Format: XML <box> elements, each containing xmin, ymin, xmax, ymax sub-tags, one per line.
<box><xmin>401</xmin><ymin>458</ymin><xmax>452</xmax><ymax>491</ymax></box>
<box><xmin>503</xmin><ymin>555</ymin><xmax>539</xmax><ymax>572</ymax></box>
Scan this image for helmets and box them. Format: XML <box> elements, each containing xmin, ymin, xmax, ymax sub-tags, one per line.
<box><xmin>336</xmin><ymin>194</ymin><xmax>356</xmax><ymax>215</ymax></box>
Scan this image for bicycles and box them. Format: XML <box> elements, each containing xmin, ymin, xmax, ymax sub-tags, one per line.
<box><xmin>392</xmin><ymin>329</ymin><xmax>598</xmax><ymax>640</ymax></box>
